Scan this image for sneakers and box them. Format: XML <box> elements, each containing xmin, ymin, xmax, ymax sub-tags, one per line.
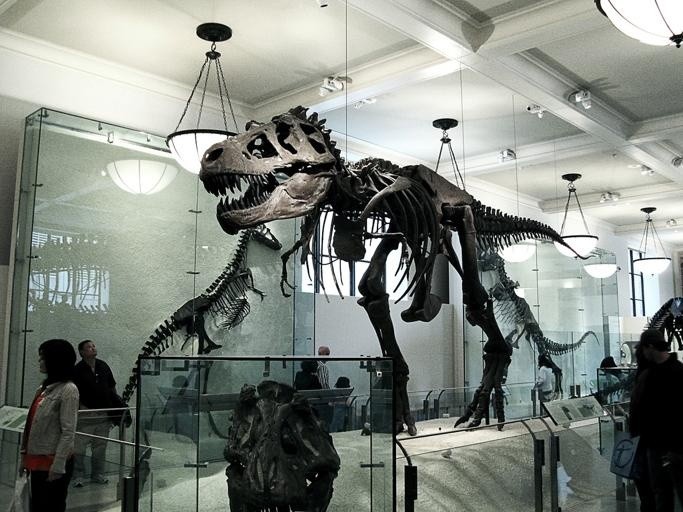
<box><xmin>71</xmin><ymin>477</ymin><xmax>83</xmax><ymax>488</ymax></box>
<box><xmin>89</xmin><ymin>473</ymin><xmax>108</xmax><ymax>485</ymax></box>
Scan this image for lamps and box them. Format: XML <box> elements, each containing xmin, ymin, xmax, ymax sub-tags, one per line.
<box><xmin>594</xmin><ymin>0</ymin><xmax>683</xmax><ymax>49</ymax></box>
<box><xmin>495</xmin><ymin>93</ymin><xmax>538</xmax><ymax>264</ymax></box>
<box><xmin>630</xmin><ymin>207</ymin><xmax>672</xmax><ymax>277</ymax></box>
<box><xmin>553</xmin><ymin>173</ymin><xmax>598</xmax><ymax>260</ymax></box>
<box><xmin>164</xmin><ymin>22</ymin><xmax>242</xmax><ymax>174</ymax></box>
<box><xmin>103</xmin><ymin>156</ymin><xmax>178</xmax><ymax>197</ymax></box>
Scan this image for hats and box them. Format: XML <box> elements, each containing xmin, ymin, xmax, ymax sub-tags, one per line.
<box><xmin>640</xmin><ymin>330</ymin><xmax>666</xmax><ymax>344</ymax></box>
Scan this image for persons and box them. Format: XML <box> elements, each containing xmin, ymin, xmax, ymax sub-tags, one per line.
<box><xmin>69</xmin><ymin>340</ymin><xmax>117</xmax><ymax>487</ymax></box>
<box><xmin>295</xmin><ymin>347</ymin><xmax>351</xmax><ymax>433</ymax></box>
<box><xmin>18</xmin><ymin>340</ymin><xmax>80</xmax><ymax>512</ymax></box>
<box><xmin>534</xmin><ymin>330</ymin><xmax>683</xmax><ymax>512</ymax></box>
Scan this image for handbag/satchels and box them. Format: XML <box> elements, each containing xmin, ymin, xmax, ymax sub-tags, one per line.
<box><xmin>14</xmin><ymin>470</ymin><xmax>32</xmax><ymax>512</ymax></box>
<box><xmin>609</xmin><ymin>430</ymin><xmax>643</xmax><ymax>481</ymax></box>
